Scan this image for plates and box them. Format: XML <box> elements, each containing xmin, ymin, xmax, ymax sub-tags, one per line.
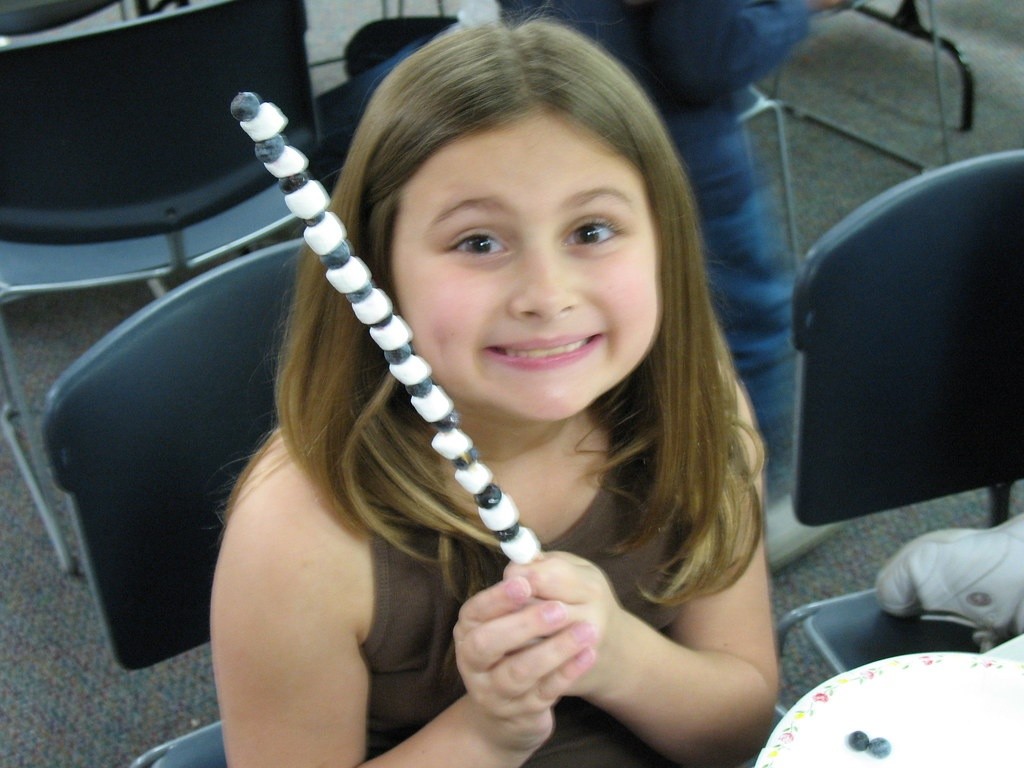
<box><xmin>761</xmin><ymin>653</ymin><xmax>1024</xmax><ymax>768</ymax></box>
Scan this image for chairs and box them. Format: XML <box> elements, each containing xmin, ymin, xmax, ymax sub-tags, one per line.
<box><xmin>774</xmin><ymin>148</ymin><xmax>1024</xmax><ymax>718</ymax></box>
<box><xmin>0</xmin><ymin>0</ymin><xmax>314</xmax><ymax>573</ymax></box>
<box><xmin>46</xmin><ymin>237</ymin><xmax>305</xmax><ymax>767</ymax></box>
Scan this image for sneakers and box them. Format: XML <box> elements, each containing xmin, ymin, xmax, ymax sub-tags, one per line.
<box><xmin>875</xmin><ymin>512</ymin><xmax>1024</xmax><ymax>652</ymax></box>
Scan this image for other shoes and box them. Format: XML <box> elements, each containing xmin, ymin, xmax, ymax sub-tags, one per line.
<box><xmin>764</xmin><ymin>493</ymin><xmax>847</xmax><ymax>572</ymax></box>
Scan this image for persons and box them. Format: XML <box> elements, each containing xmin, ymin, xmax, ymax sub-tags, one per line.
<box><xmin>209</xmin><ymin>18</ymin><xmax>779</xmax><ymax>768</ymax></box>
<box><xmin>493</xmin><ymin>0</ymin><xmax>848</xmax><ymax>570</ymax></box>
